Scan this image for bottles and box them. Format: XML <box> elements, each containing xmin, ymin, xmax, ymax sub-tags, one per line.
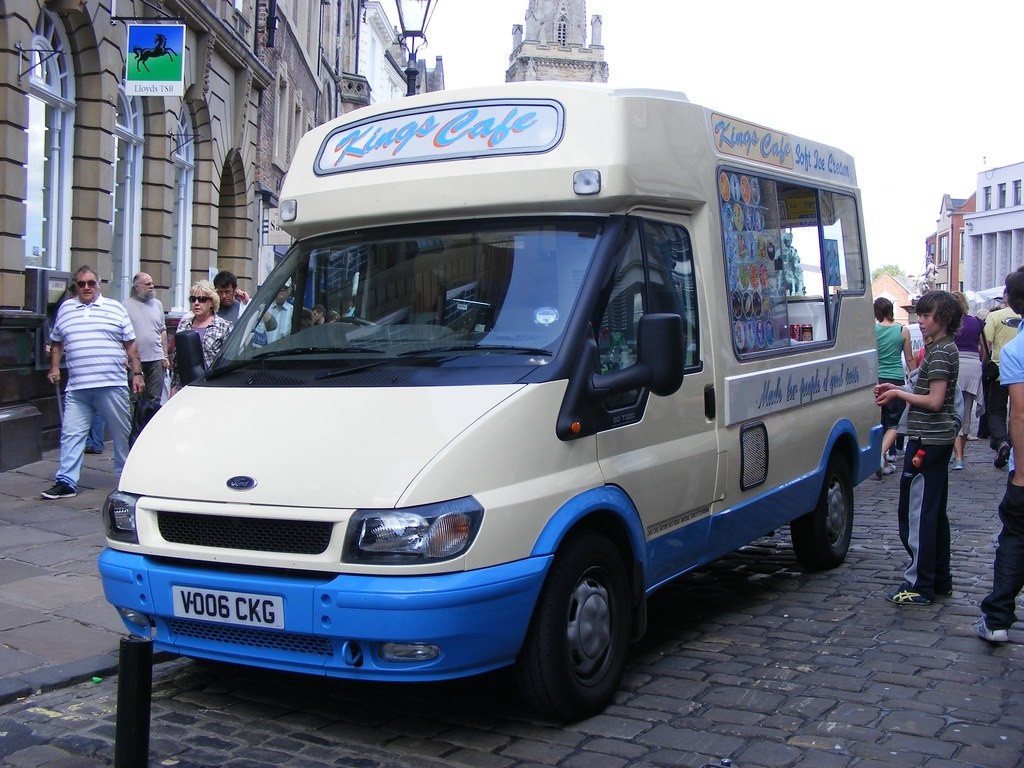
<box><xmin>601</xmin><ymin>342</ymin><xmax>637</xmax><ymax>404</ymax></box>
<box><xmin>912</xmin><ymin>449</ymin><xmax>925</xmax><ymax>468</ymax></box>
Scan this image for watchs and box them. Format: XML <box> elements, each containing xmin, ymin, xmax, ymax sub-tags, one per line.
<box><xmin>134</xmin><ymin>372</ymin><xmax>145</xmax><ymax>376</ymax></box>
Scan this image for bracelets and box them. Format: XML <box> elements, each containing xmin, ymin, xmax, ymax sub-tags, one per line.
<box><xmin>164</xmin><ymin>356</ymin><xmax>169</xmax><ymax>358</ymax></box>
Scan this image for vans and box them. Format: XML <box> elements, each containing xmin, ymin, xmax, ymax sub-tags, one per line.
<box><xmin>95</xmin><ymin>78</ymin><xmax>884</xmax><ymax>724</ymax></box>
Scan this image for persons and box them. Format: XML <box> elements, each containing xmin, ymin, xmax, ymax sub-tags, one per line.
<box><xmin>872</xmin><ymin>289</ymin><xmax>961</xmax><ymax>605</ymax></box>
<box><xmin>168</xmin><ymin>277</ymin><xmax>235</xmax><ymax>397</ymax></box>
<box><xmin>323</xmin><ymin>310</ymin><xmax>340</xmax><ymax>324</ymax></box>
<box><xmin>214</xmin><ymin>267</ymin><xmax>278</xmax><ymax>349</ymax></box>
<box><xmin>40</xmin><ymin>264</ymin><xmax>146</xmax><ymax>496</ymax></box>
<box><xmin>265</xmin><ymin>277</ymin><xmax>293</xmax><ymax>343</ymax></box>
<box><xmin>312</xmin><ymin>304</ymin><xmax>326</xmax><ymax>326</ymax></box>
<box><xmin>916</xmin><ymin>256</ymin><xmax>940</xmax><ymax>298</ymax></box>
<box><xmin>300</xmin><ymin>301</ymin><xmax>314</xmax><ymax>330</ymax></box>
<box><xmin>123</xmin><ymin>271</ymin><xmax>170</xmax><ymax>450</ymax></box>
<box><xmin>874</xmin><ymin>297</ymin><xmax>933</xmax><ymax>474</ymax></box>
<box><xmin>975</xmin><ymin>264</ymin><xmax>1024</xmax><ymax>642</ymax></box>
<box><xmin>345</xmin><ymin>295</ymin><xmax>357</xmax><ymax>314</ymax></box>
<box><xmin>951</xmin><ymin>290</ymin><xmax>987</xmax><ymax>470</ymax></box>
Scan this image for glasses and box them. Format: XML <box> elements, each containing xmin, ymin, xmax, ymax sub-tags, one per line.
<box><xmin>75</xmin><ymin>280</ymin><xmax>99</xmax><ymax>287</ymax></box>
<box><xmin>189</xmin><ymin>294</ymin><xmax>213</xmax><ymax>303</ymax></box>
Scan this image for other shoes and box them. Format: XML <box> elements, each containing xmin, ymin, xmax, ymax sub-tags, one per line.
<box><xmin>84</xmin><ymin>446</ymin><xmax>100</xmax><ymax>454</ymax></box>
<box><xmin>883</xmin><ymin>463</ymin><xmax>896</xmax><ymax>474</ymax></box>
<box><xmin>967</xmin><ymin>433</ymin><xmax>979</xmax><ymax>440</ymax></box>
<box><xmin>993</xmin><ymin>441</ymin><xmax>1011</xmax><ymax>468</ymax></box>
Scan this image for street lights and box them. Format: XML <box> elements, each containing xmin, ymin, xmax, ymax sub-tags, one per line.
<box><xmin>394</xmin><ymin>0</ymin><xmax>440</xmax><ymax>97</ymax></box>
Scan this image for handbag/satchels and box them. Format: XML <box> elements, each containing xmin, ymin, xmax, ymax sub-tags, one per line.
<box><xmin>987</xmin><ymin>360</ymin><xmax>1000</xmax><ymax>382</ymax></box>
<box><xmin>160</xmin><ymin>367</ymin><xmax>172</xmax><ymax>406</ymax></box>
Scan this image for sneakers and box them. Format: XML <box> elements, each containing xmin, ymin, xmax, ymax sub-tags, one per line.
<box><xmin>974</xmin><ymin>612</ymin><xmax>1009</xmax><ymax>641</ymax></box>
<box><xmin>886</xmin><ymin>454</ymin><xmax>897</xmax><ymax>462</ymax></box>
<box><xmin>886</xmin><ymin>582</ymin><xmax>933</xmax><ymax>606</ymax></box>
<box><xmin>895</xmin><ymin>448</ymin><xmax>907</xmax><ymax>458</ymax></box>
<box><xmin>41</xmin><ymin>479</ymin><xmax>76</xmax><ymax>498</ymax></box>
<box><xmin>952</xmin><ymin>459</ymin><xmax>963</xmax><ymax>469</ymax></box>
